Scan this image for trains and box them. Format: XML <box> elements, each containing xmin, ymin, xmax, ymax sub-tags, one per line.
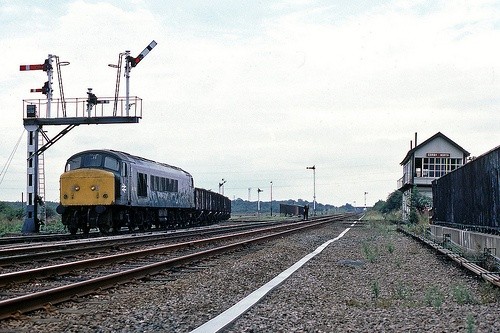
<box><xmin>56</xmin><ymin>148</ymin><xmax>232</xmax><ymax>237</ymax></box>
